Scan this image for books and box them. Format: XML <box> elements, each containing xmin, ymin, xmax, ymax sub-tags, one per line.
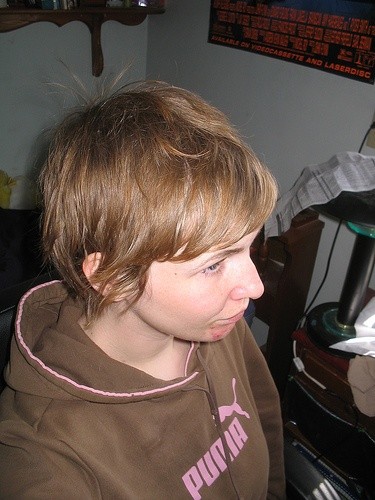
<box><xmin>282</xmin><ymin>420</ymin><xmax>366</xmax><ymax>500</ymax></box>
<box><xmin>0</xmin><ymin>0</ymin><xmax>154</xmax><ymax>11</ymax></box>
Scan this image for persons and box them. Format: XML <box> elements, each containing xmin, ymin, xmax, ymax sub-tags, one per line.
<box><xmin>0</xmin><ymin>56</ymin><xmax>286</xmax><ymax>500</ymax></box>
